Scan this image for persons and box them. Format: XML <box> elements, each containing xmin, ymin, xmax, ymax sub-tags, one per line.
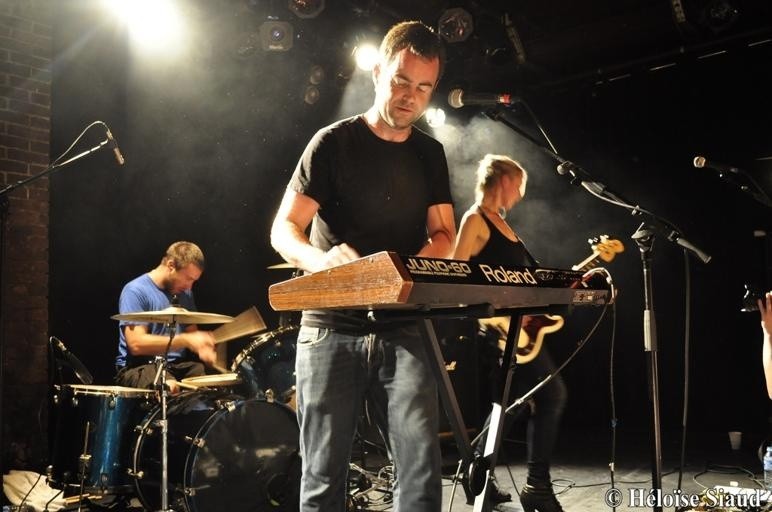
<box><xmin>269</xmin><ymin>21</ymin><xmax>459</xmax><ymax>512</ymax></box>
<box><xmin>757</xmin><ymin>292</ymin><xmax>772</xmax><ymax>401</ymax></box>
<box><xmin>449</xmin><ymin>155</ymin><xmax>567</xmax><ymax>512</ymax></box>
<box><xmin>113</xmin><ymin>240</ymin><xmax>215</xmax><ymax>469</ymax></box>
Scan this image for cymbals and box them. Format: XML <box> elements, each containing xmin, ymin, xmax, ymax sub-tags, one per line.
<box><xmin>111</xmin><ymin>307</ymin><xmax>236</xmax><ymax>324</ymax></box>
<box><xmin>268</xmin><ymin>263</ymin><xmax>297</xmax><ymax>269</ymax></box>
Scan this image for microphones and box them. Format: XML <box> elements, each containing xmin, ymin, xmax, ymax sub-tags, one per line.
<box><xmin>102</xmin><ymin>124</ymin><xmax>125</xmax><ymax>165</ymax></box>
<box><xmin>57</xmin><ymin>342</ymin><xmax>94</xmax><ymax>385</ymax></box>
<box><xmin>448</xmin><ymin>89</ymin><xmax>520</xmax><ymax>109</ymax></box>
<box><xmin>693</xmin><ymin>156</ymin><xmax>740</xmax><ymax>174</ymax></box>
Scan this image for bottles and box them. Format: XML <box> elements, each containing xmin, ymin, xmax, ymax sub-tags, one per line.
<box><xmin>763</xmin><ymin>446</ymin><xmax>772</xmax><ymax>488</ymax></box>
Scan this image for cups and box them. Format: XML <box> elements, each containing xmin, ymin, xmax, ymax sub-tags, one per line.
<box><xmin>729</xmin><ymin>432</ymin><xmax>742</xmax><ymax>448</ymax></box>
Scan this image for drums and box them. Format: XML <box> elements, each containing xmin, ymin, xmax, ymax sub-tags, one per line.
<box><xmin>181</xmin><ymin>372</ymin><xmax>242</xmax><ymax>392</ymax></box>
<box><xmin>48</xmin><ymin>384</ymin><xmax>159</xmax><ymax>495</ymax></box>
<box><xmin>129</xmin><ymin>391</ymin><xmax>301</xmax><ymax>512</ymax></box>
<box><xmin>232</xmin><ymin>325</ymin><xmax>300</xmax><ymax>409</ymax></box>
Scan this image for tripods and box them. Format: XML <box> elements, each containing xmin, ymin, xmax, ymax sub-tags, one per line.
<box><xmin>348</xmin><ymin>418</ymin><xmax>392</xmax><ymax>498</ymax></box>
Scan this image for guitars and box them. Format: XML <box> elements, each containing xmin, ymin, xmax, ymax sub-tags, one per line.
<box><xmin>478</xmin><ymin>235</ymin><xmax>625</xmax><ymax>364</ymax></box>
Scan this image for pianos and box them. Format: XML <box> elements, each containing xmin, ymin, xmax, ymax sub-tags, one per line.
<box><xmin>269</xmin><ymin>251</ymin><xmax>618</xmax><ymax>310</ymax></box>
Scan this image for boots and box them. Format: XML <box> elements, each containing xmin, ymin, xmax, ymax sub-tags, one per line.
<box><xmin>517</xmin><ymin>460</ymin><xmax>564</xmax><ymax>512</ymax></box>
<box><xmin>459</xmin><ymin>463</ymin><xmax>512</xmax><ymax>505</ymax></box>
